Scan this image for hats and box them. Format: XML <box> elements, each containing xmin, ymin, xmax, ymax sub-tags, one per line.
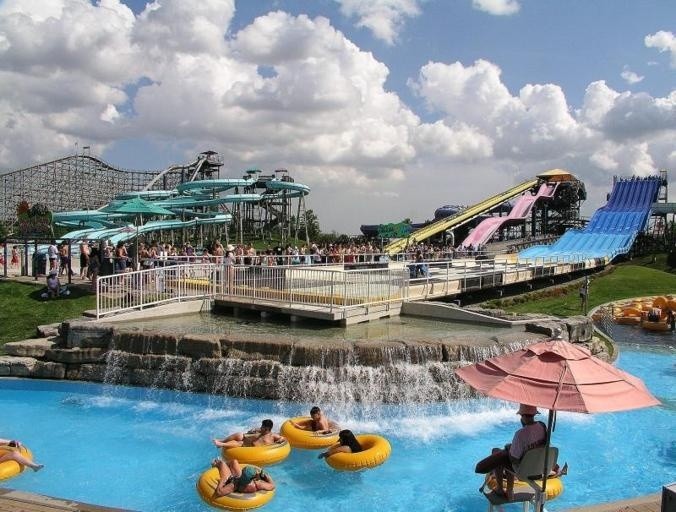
<box><xmin>516</xmin><ymin>404</ymin><xmax>540</xmax><ymax>415</ymax></box>
<box><xmin>238</xmin><ymin>466</ymin><xmax>256</xmax><ymax>484</ymax></box>
<box><xmin>225</xmin><ymin>244</ymin><xmax>235</xmax><ymax>252</ymax></box>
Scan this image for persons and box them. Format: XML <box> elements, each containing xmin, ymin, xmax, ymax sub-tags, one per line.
<box><xmin>80</xmin><ymin>239</ymin><xmax>100</xmax><ymax>280</ymax></box>
<box><xmin>211</xmin><ymin>456</ymin><xmax>276</xmax><ymax>497</ymax></box>
<box><xmin>288</xmin><ymin>405</ymin><xmax>330</xmax><ymax>433</ymax></box>
<box><xmin>644</xmin><ymin>304</ymin><xmax>663</xmax><ymax>322</ymax></box>
<box><xmin>138</xmin><ymin>239</ymin><xmax>380</xmax><ymax>265</ymax></box>
<box><xmin>486</xmin><ymin>403</ymin><xmax>549</xmax><ymax>501</ymax></box>
<box><xmin>414</xmin><ymin>249</ymin><xmax>425</xmax><ymax>278</ymax></box>
<box><xmin>315</xmin><ymin>429</ymin><xmax>362</xmax><ymax>461</ymax></box>
<box><xmin>11</xmin><ymin>246</ymin><xmax>19</xmax><ymax>268</ymax></box>
<box><xmin>0</xmin><ymin>436</ymin><xmax>45</xmax><ymax>472</ymax></box>
<box><xmin>209</xmin><ymin>417</ymin><xmax>286</xmax><ymax>448</ymax></box>
<box><xmin>49</xmin><ymin>240</ymin><xmax>68</xmax><ymax>297</ymax></box>
<box><xmin>404</xmin><ymin>238</ymin><xmax>482</xmax><ymax>260</ymax></box>
<box><xmin>666</xmin><ymin>307</ymin><xmax>676</xmax><ymax>333</ymax></box>
<box><xmin>104</xmin><ymin>240</ymin><xmax>130</xmax><ymax>269</ymax></box>
<box><xmin>579</xmin><ymin>279</ymin><xmax>593</xmax><ymax>314</ymax></box>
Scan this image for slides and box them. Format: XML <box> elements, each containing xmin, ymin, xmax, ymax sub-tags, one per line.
<box><xmin>27</xmin><ymin>161</ymin><xmax>310</xmax><ymax>254</ymax></box>
<box><xmin>457</xmin><ymin>181</ymin><xmax>561</xmax><ymax>250</ymax></box>
<box><xmin>516</xmin><ymin>178</ymin><xmax>660</xmax><ymax>266</ymax></box>
<box><xmin>382</xmin><ymin>180</ymin><xmax>540</xmax><ymax>256</ymax></box>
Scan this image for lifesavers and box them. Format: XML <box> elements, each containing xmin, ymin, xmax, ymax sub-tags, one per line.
<box><xmin>220</xmin><ymin>431</ymin><xmax>291</xmax><ymax>466</ymax></box>
<box><xmin>279</xmin><ymin>416</ymin><xmax>344</xmax><ymax>450</ymax></box>
<box><xmin>0</xmin><ymin>439</ymin><xmax>32</xmax><ymax>481</ymax></box>
<box><xmin>325</xmin><ymin>433</ymin><xmax>391</xmax><ymax>471</ymax></box>
<box><xmin>594</xmin><ymin>296</ymin><xmax>676</xmax><ymax>330</ymax></box>
<box><xmin>484</xmin><ymin>466</ymin><xmax>564</xmax><ymax>501</ymax></box>
<box><xmin>196</xmin><ymin>461</ymin><xmax>274</xmax><ymax>510</ymax></box>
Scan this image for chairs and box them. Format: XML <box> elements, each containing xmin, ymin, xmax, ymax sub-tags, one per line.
<box><xmin>500</xmin><ymin>448</ymin><xmax>558</xmax><ymax>482</ymax></box>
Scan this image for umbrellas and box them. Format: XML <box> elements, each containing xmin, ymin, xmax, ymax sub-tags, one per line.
<box><xmin>445</xmin><ymin>326</ymin><xmax>666</xmax><ymax>497</ymax></box>
